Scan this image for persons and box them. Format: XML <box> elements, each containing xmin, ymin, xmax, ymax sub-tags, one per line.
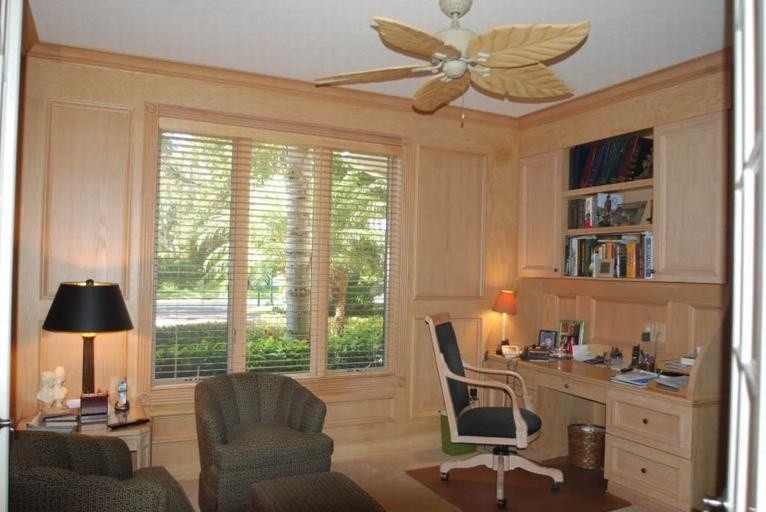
<box><xmin>541</xmin><ymin>336</ymin><xmax>552</xmax><ymax>350</ymax></box>
<box><xmin>36</xmin><ymin>371</ymin><xmax>55</xmax><ymax>404</ymax></box>
<box><xmin>52</xmin><ymin>367</ymin><xmax>68</xmax><ymax>400</ymax></box>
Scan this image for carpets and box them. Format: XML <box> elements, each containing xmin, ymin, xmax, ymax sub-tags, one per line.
<box><xmin>405</xmin><ymin>458</ymin><xmax>631</xmax><ymax>512</ymax></box>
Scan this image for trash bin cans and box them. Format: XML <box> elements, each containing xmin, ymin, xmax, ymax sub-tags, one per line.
<box><xmin>568</xmin><ymin>425</ymin><xmax>605</xmax><ymax>470</ymax></box>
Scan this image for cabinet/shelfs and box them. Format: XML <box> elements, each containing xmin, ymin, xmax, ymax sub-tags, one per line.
<box><xmin>562</xmin><ymin>130</ymin><xmax>654</xmax><ymax>282</ymax></box>
<box><xmin>518</xmin><ymin>149</ymin><xmax>562</xmax><ymax>279</ymax></box>
<box><xmin>654</xmin><ymin>111</ymin><xmax>730</xmax><ymax>284</ymax></box>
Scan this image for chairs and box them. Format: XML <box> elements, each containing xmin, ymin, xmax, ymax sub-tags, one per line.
<box><xmin>193</xmin><ymin>373</ymin><xmax>333</xmax><ymax>511</ymax></box>
<box><xmin>425</xmin><ymin>312</ymin><xmax>564</xmax><ymax>507</ymax></box>
<box><xmin>9</xmin><ymin>430</ymin><xmax>194</xmax><ymax>512</ymax></box>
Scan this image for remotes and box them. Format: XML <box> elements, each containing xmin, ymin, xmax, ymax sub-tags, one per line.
<box><xmin>107</xmin><ymin>417</ymin><xmax>150</xmax><ymax>429</ymax></box>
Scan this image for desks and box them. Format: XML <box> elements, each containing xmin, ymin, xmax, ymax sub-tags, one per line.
<box><xmin>26</xmin><ymin>412</ymin><xmax>150</xmax><ymax>469</ymax></box>
<box><xmin>484</xmin><ymin>303</ymin><xmax>723</xmax><ymax>512</ymax></box>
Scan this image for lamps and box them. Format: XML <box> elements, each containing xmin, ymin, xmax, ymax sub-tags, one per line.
<box><xmin>44</xmin><ymin>280</ymin><xmax>133</xmax><ymax>394</ymax></box>
<box><xmin>492</xmin><ymin>291</ymin><xmax>518</xmax><ymax>355</ymax></box>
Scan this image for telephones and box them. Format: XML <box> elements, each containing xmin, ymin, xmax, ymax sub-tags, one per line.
<box><xmin>629</xmin><ymin>343</ymin><xmax>640</xmax><ymax>369</ymax></box>
<box><xmin>114</xmin><ymin>375</ymin><xmax>129</xmax><ymax>413</ymax></box>
<box><xmin>520</xmin><ymin>348</ymin><xmax>551</xmax><ymax>359</ymax></box>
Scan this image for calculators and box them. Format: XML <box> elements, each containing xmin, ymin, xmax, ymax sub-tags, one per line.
<box><xmin>584</xmin><ymin>355</ymin><xmax>604</xmax><ymax>365</ymax></box>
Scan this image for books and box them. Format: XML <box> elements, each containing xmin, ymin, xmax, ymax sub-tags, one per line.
<box><xmin>609</xmin><ymin>352</ymin><xmax>697</xmax><ymax>397</ymax></box>
<box><xmin>564</xmin><ymin>134</ymin><xmax>653</xmax><ymax>280</ymax></box>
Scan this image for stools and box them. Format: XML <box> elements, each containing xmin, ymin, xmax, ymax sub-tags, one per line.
<box><xmin>251</xmin><ymin>473</ymin><xmax>386</xmax><ymax>512</ymax></box>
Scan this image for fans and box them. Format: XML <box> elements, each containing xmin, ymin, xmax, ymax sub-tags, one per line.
<box><xmin>315</xmin><ymin>0</ymin><xmax>588</xmax><ymax>121</ymax></box>
